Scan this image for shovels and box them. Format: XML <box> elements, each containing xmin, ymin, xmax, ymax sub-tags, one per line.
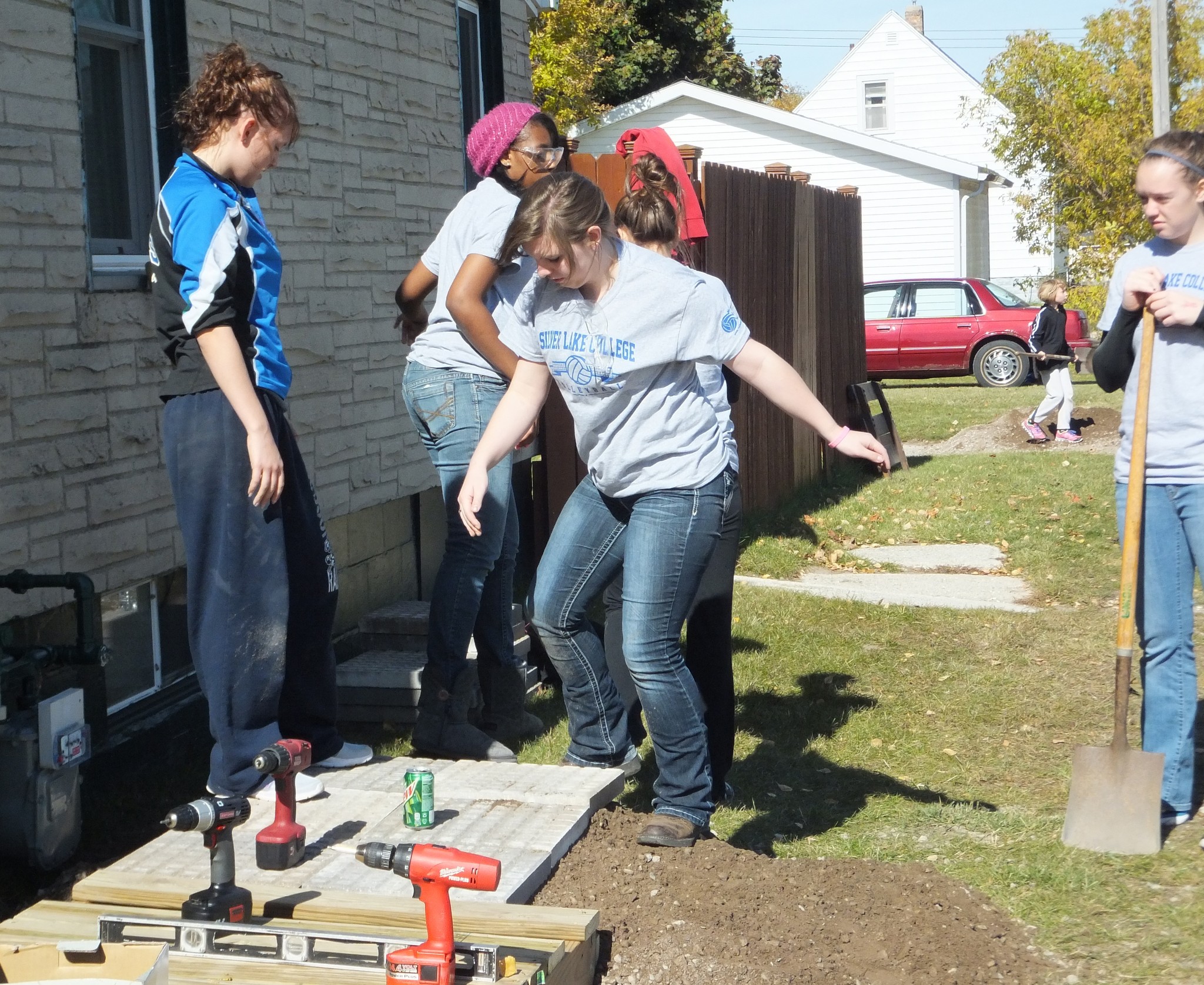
<box><xmin>1061</xmin><ymin>298</ymin><xmax>1165</xmax><ymax>857</ymax></box>
<box><xmin>1014</xmin><ymin>345</ymin><xmax>1095</xmax><ymax>375</ymax></box>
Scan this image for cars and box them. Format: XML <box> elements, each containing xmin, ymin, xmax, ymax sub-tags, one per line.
<box><xmin>863</xmin><ymin>276</ymin><xmax>1098</xmax><ymax>389</ymax></box>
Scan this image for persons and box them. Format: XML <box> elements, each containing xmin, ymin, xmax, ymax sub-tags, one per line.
<box><xmin>1021</xmin><ymin>278</ymin><xmax>1083</xmax><ymax>443</ymax></box>
<box><xmin>456</xmin><ymin>173</ymin><xmax>893</xmax><ymax>849</ymax></box>
<box><xmin>393</xmin><ymin>103</ymin><xmax>566</xmax><ymax>762</ymax></box>
<box><xmin>1090</xmin><ymin>132</ymin><xmax>1204</xmax><ymax>827</ymax></box>
<box><xmin>603</xmin><ymin>151</ymin><xmax>746</xmax><ymax>814</ymax></box>
<box><xmin>146</xmin><ymin>43</ymin><xmax>373</xmax><ymax>800</ymax></box>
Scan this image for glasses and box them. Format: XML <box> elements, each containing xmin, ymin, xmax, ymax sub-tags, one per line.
<box><xmin>511</xmin><ymin>145</ymin><xmax>564</xmax><ymax>173</ymax></box>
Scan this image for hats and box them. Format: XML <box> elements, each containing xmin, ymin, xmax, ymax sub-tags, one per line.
<box><xmin>466</xmin><ymin>101</ymin><xmax>541</xmax><ymax>177</ymax></box>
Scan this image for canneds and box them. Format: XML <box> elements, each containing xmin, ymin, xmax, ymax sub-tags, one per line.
<box><xmin>403</xmin><ymin>766</ymin><xmax>435</xmax><ymax>830</ymax></box>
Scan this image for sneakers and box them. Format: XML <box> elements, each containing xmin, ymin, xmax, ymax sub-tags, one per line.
<box><xmin>1021</xmin><ymin>418</ymin><xmax>1047</xmax><ymax>442</ymax></box>
<box><xmin>1055</xmin><ymin>429</ymin><xmax>1083</xmax><ymax>443</ymax></box>
<box><xmin>312</xmin><ymin>742</ymin><xmax>373</xmax><ymax>768</ymax></box>
<box><xmin>206</xmin><ymin>771</ymin><xmax>324</xmax><ymax>803</ymax></box>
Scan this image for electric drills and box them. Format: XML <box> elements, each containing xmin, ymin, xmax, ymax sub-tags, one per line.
<box><xmin>158</xmin><ymin>793</ymin><xmax>253</xmax><ymax>934</ymax></box>
<box><xmin>352</xmin><ymin>840</ymin><xmax>501</xmax><ymax>985</ymax></box>
<box><xmin>252</xmin><ymin>738</ymin><xmax>314</xmax><ymax>871</ymax></box>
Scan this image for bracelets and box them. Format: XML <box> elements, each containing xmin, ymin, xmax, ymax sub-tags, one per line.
<box><xmin>827</xmin><ymin>425</ymin><xmax>850</xmax><ymax>450</ymax></box>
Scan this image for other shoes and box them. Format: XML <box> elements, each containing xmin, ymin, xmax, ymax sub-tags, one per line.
<box><xmin>1159</xmin><ymin>811</ymin><xmax>1189</xmax><ymax>826</ymax></box>
<box><xmin>636</xmin><ymin>810</ymin><xmax>711</xmax><ymax>847</ymax></box>
<box><xmin>555</xmin><ymin>753</ymin><xmax>643</xmax><ymax>777</ymax></box>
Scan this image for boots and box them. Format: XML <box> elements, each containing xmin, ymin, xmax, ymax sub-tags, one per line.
<box><xmin>476</xmin><ymin>665</ymin><xmax>545</xmax><ymax>738</ymax></box>
<box><xmin>411</xmin><ymin>663</ymin><xmax>518</xmax><ymax>763</ymax></box>
